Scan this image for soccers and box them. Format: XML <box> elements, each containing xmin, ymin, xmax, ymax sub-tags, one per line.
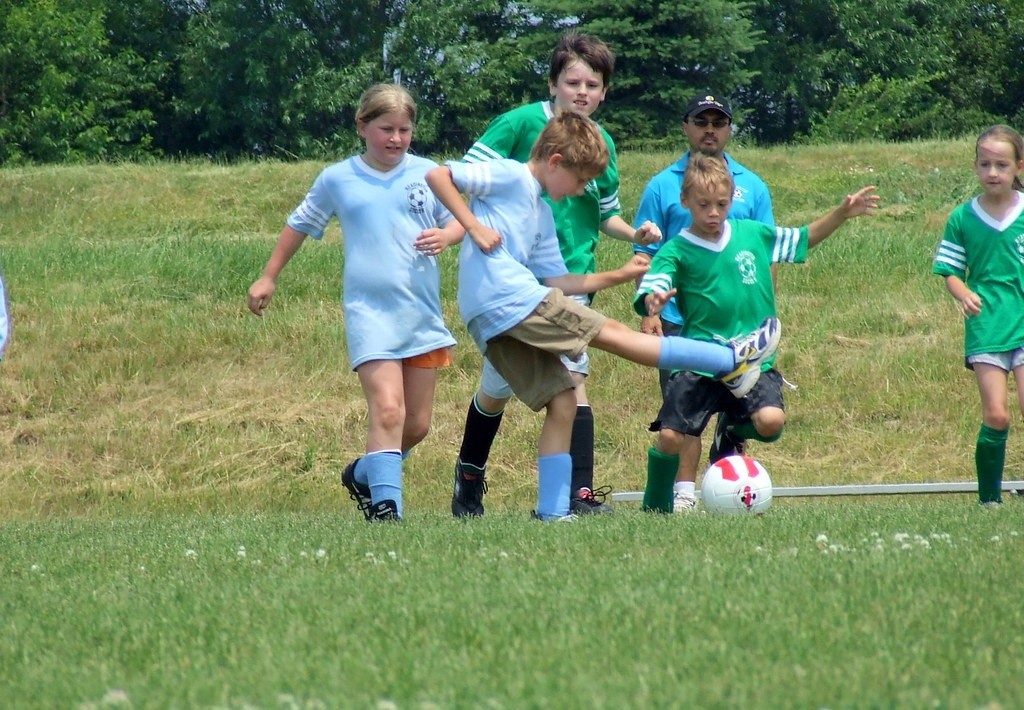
<box><xmin>701</xmin><ymin>454</ymin><xmax>774</xmax><ymax>518</ymax></box>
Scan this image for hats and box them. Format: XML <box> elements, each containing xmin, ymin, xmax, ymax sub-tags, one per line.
<box><xmin>684</xmin><ymin>93</ymin><xmax>732</xmax><ymax>127</ymax></box>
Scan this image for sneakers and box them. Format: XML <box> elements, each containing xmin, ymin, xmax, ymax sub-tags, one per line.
<box><xmin>451</xmin><ymin>456</ymin><xmax>488</xmax><ymax>519</ymax></box>
<box><xmin>720</xmin><ymin>317</ymin><xmax>782</xmax><ymax>399</ymax></box>
<box><xmin>340</xmin><ymin>458</ymin><xmax>399</xmax><ymax>523</ymax></box>
<box><xmin>570</xmin><ymin>484</ymin><xmax>615</xmax><ymax>519</ymax></box>
<box><xmin>709</xmin><ymin>410</ymin><xmax>747</xmax><ymax>467</ymax></box>
<box><xmin>672</xmin><ymin>489</ymin><xmax>695</xmax><ymax>513</ymax></box>
<box><xmin>531</xmin><ymin>510</ymin><xmax>578</xmax><ymax>522</ymax></box>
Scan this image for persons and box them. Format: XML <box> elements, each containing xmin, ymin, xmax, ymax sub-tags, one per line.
<box><xmin>424</xmin><ymin>113</ymin><xmax>781</xmax><ymax>522</ymax></box>
<box><xmin>932</xmin><ymin>125</ymin><xmax>1024</xmax><ymax>511</ymax></box>
<box><xmin>632</xmin><ymin>151</ymin><xmax>882</xmax><ymax>514</ymax></box>
<box><xmin>452</xmin><ymin>33</ymin><xmax>663</xmax><ymax>516</ymax></box>
<box><xmin>247</xmin><ymin>84</ymin><xmax>467</xmax><ymax>521</ymax></box>
<box><xmin>633</xmin><ymin>91</ymin><xmax>777</xmax><ymax>514</ymax></box>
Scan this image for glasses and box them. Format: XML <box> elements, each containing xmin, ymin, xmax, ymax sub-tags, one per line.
<box><xmin>688</xmin><ymin>116</ymin><xmax>729</xmax><ymax>128</ymax></box>
